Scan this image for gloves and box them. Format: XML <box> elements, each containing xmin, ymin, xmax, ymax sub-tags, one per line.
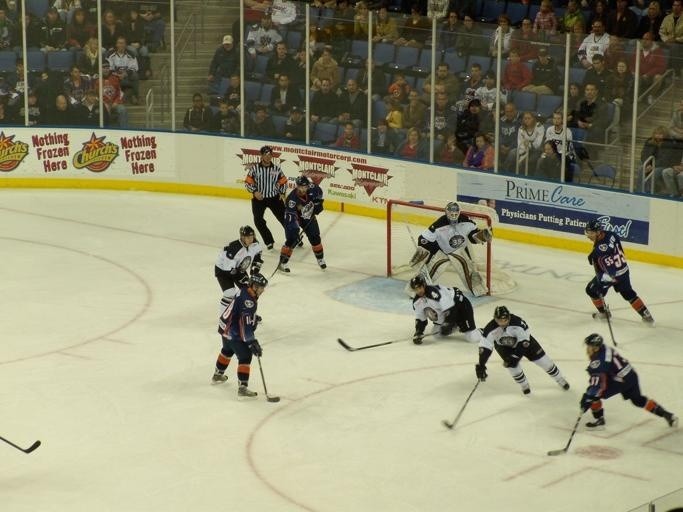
<box><xmin>413</xmin><ymin>333</ymin><xmax>424</xmax><ymax>344</ymax></box>
<box><xmin>441</xmin><ymin>321</ymin><xmax>451</xmax><ymax>334</ymax></box>
<box><xmin>476</xmin><ymin>364</ymin><xmax>487</xmax><ymax>380</ymax></box>
<box><xmin>581</xmin><ymin>393</ymin><xmax>594</xmax><ymax>411</ymax></box>
<box><xmin>248</xmin><ymin>340</ymin><xmax>262</xmax><ymax>357</ymax></box>
<box><xmin>504</xmin><ymin>354</ymin><xmax>519</xmax><ymax>367</ymax></box>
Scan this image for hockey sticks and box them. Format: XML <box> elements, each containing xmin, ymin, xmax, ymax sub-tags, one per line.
<box><xmin>547</xmin><ymin>409</ymin><xmax>584</xmax><ymax>456</ymax></box>
<box><xmin>443</xmin><ymin>378</ymin><xmax>484</xmax><ymax>429</ymax></box>
<box><xmin>270</xmin><ymin>215</ymin><xmax>317</xmax><ymax>276</ymax></box>
<box><xmin>257</xmin><ymin>353</ymin><xmax>281</xmax><ymax>402</ymax></box>
<box><xmin>592</xmin><ymin>264</ymin><xmax>617</xmax><ymax>349</ymax></box>
<box><xmin>338</xmin><ymin>333</ymin><xmax>444</xmax><ymax>351</ymax></box>
<box><xmin>0</xmin><ymin>434</ymin><xmax>40</xmax><ymax>453</ymax></box>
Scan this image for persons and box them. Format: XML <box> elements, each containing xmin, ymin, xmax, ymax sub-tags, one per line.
<box><xmin>185</xmin><ymin>0</ymin><xmax>681</xmax><ymax>183</ymax></box>
<box><xmin>637</xmin><ymin>126</ymin><xmax>681</xmax><ymax>198</ymax></box>
<box><xmin>579</xmin><ymin>334</ymin><xmax>678</xmax><ymax>429</ymax></box>
<box><xmin>476</xmin><ymin>306</ymin><xmax>570</xmax><ymax>394</ymax></box>
<box><xmin>210</xmin><ymin>273</ymin><xmax>268</xmax><ymax>398</ymax></box>
<box><xmin>244</xmin><ymin>145</ymin><xmax>304</xmax><ymax>249</ymax></box>
<box><xmin>585</xmin><ymin>218</ymin><xmax>656</xmax><ymax>326</ymax></box>
<box><xmin>2</xmin><ymin>0</ymin><xmax>178</xmax><ymax>125</ymax></box>
<box><xmin>405</xmin><ymin>202</ymin><xmax>493</xmax><ymax>303</ymax></box>
<box><xmin>409</xmin><ymin>274</ymin><xmax>484</xmax><ymax>344</ymax></box>
<box><xmin>278</xmin><ymin>175</ymin><xmax>326</xmax><ymax>272</ymax></box>
<box><xmin>215</xmin><ymin>225</ymin><xmax>264</xmax><ymax>335</ymax></box>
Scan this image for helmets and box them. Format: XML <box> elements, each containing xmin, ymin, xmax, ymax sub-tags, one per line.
<box><xmin>584</xmin><ymin>219</ymin><xmax>601</xmax><ymax>237</ymax></box>
<box><xmin>445</xmin><ymin>202</ymin><xmax>460</xmax><ymax>220</ymax></box>
<box><xmin>296</xmin><ymin>176</ymin><xmax>308</xmax><ymax>186</ymax></box>
<box><xmin>585</xmin><ymin>334</ymin><xmax>603</xmax><ymax>346</ymax></box>
<box><xmin>250</xmin><ymin>274</ymin><xmax>268</xmax><ymax>286</ymax></box>
<box><xmin>411</xmin><ymin>275</ymin><xmax>427</xmax><ymax>288</ymax></box>
<box><xmin>494</xmin><ymin>306</ymin><xmax>509</xmax><ymax>318</ymax></box>
<box><xmin>240</xmin><ymin>226</ymin><xmax>254</xmax><ymax>236</ymax></box>
<box><xmin>260</xmin><ymin>147</ymin><xmax>271</xmax><ymax>155</ymax></box>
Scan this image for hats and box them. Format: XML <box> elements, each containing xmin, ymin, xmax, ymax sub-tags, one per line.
<box><xmin>223</xmin><ymin>35</ymin><xmax>233</xmax><ymax>45</ymax></box>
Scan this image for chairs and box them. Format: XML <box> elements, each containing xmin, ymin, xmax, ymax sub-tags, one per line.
<box><xmin>0</xmin><ymin>0</ymin><xmax>177</xmax><ymax>126</ymax></box>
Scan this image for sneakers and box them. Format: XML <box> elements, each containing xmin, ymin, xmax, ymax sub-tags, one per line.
<box><xmin>522</xmin><ymin>384</ymin><xmax>530</xmax><ymax>394</ymax></box>
<box><xmin>278</xmin><ymin>263</ymin><xmax>290</xmax><ymax>272</ymax></box>
<box><xmin>668</xmin><ymin>413</ymin><xmax>674</xmax><ymax>426</ymax></box>
<box><xmin>317</xmin><ymin>259</ymin><xmax>326</xmax><ymax>268</ymax></box>
<box><xmin>593</xmin><ymin>312</ymin><xmax>611</xmax><ymax>318</ymax></box>
<box><xmin>642</xmin><ymin>316</ymin><xmax>654</xmax><ymax>321</ymax></box>
<box><xmin>213</xmin><ymin>375</ymin><xmax>257</xmax><ymax>396</ymax></box>
<box><xmin>586</xmin><ymin>417</ymin><xmax>604</xmax><ymax>426</ymax></box>
<box><xmin>268</xmin><ymin>243</ymin><xmax>273</xmax><ymax>250</ymax></box>
<box><xmin>557</xmin><ymin>377</ymin><xmax>569</xmax><ymax>389</ymax></box>
